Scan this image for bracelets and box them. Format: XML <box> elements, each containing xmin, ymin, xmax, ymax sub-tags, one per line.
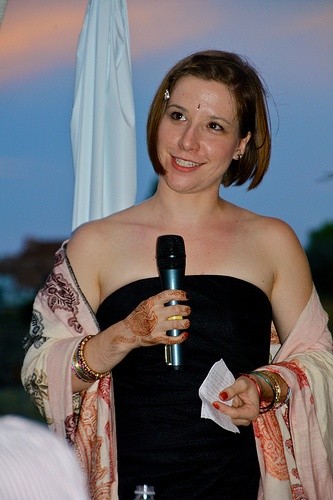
<box><xmin>69</xmin><ymin>334</ymin><xmax>110</xmax><ymax>385</ymax></box>
<box><xmin>240</xmin><ymin>371</ymin><xmax>292</xmax><ymax>414</ymax></box>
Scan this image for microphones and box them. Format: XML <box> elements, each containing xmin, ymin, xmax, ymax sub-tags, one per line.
<box><xmin>156</xmin><ymin>234</ymin><xmax>186</xmax><ymax>371</ymax></box>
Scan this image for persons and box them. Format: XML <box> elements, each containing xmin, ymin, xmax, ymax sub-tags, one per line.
<box><xmin>19</xmin><ymin>49</ymin><xmax>332</xmax><ymax>500</ymax></box>
